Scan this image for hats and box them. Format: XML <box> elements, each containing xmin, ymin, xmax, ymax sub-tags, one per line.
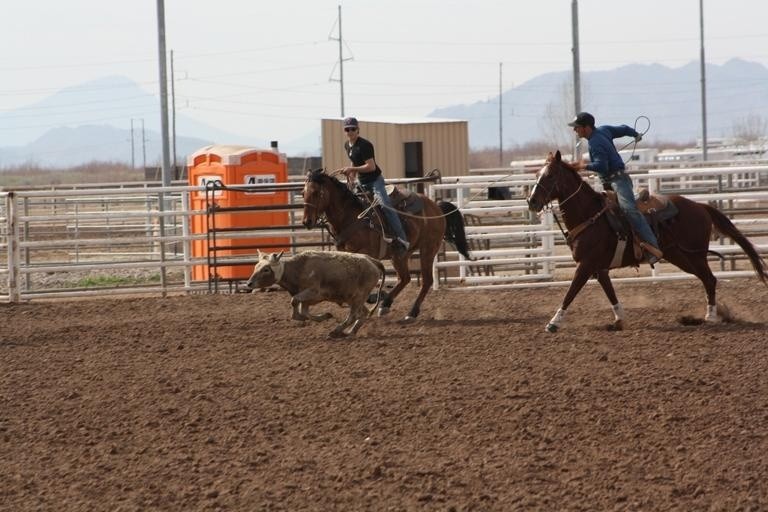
<box><xmin>343</xmin><ymin>117</ymin><xmax>358</xmax><ymax>128</ymax></box>
<box><xmin>568</xmin><ymin>113</ymin><xmax>594</xmax><ymax>128</ymax></box>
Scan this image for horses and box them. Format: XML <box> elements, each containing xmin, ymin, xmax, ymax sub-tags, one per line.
<box><xmin>300</xmin><ymin>168</ymin><xmax>472</xmax><ymax>322</ymax></box>
<box><xmin>526</xmin><ymin>149</ymin><xmax>768</xmax><ymax>335</ymax></box>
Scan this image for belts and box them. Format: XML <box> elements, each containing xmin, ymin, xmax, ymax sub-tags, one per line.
<box><xmin>600</xmin><ymin>169</ymin><xmax>622</xmax><ymax>184</ymax></box>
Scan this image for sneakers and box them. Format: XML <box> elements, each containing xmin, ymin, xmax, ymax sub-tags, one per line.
<box><xmin>391</xmin><ymin>231</ymin><xmax>406</xmax><ymax>249</ymax></box>
<box><xmin>649</xmin><ymin>256</ymin><xmax>657</xmax><ymax>263</ymax></box>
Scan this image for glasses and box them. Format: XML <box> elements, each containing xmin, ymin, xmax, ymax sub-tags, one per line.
<box><xmin>344</xmin><ymin>128</ymin><xmax>355</xmax><ymax>132</ymax></box>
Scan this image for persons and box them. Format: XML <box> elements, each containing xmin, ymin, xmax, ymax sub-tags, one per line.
<box><xmin>342</xmin><ymin>117</ymin><xmax>408</xmax><ymax>257</ymax></box>
<box><xmin>567</xmin><ymin>112</ymin><xmax>663</xmax><ymax>264</ymax></box>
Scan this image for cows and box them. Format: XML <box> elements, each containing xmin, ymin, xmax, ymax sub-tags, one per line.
<box><xmin>245</xmin><ymin>246</ymin><xmax>386</xmax><ymax>342</ymax></box>
